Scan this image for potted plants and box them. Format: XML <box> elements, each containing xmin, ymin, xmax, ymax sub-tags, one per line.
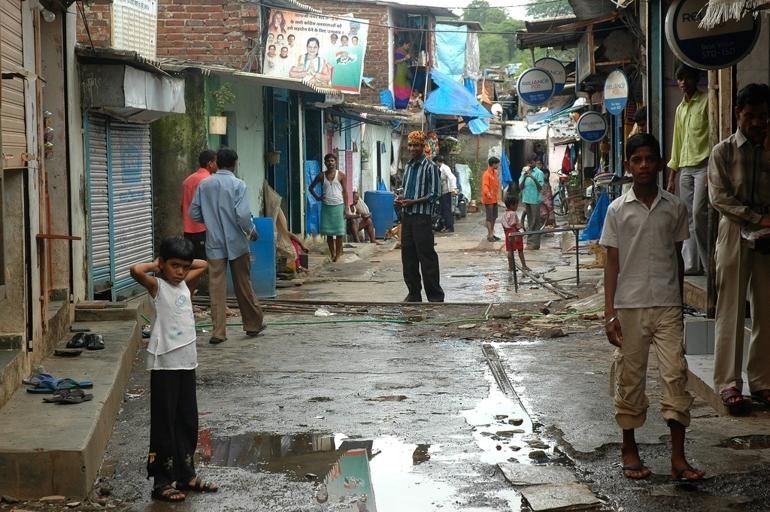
<box><xmin>207</xmin><ymin>80</ymin><xmax>237</xmax><ymax>136</ymax></box>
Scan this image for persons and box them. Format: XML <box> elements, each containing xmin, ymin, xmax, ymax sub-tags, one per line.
<box><xmin>316</xmin><ymin>483</ymin><xmax>330</xmax><ymax>504</ymax></box>
<box><xmin>501</xmin><ymin>197</ymin><xmax>531</xmax><ymax>271</ymax></box>
<box><xmin>483</xmin><ymin>156</ymin><xmax>502</xmax><ymax>241</ymax></box>
<box><xmin>662</xmin><ymin>63</ymin><xmax>717</xmax><ymax>279</ymax></box>
<box><xmin>394</xmin><ymin>132</ymin><xmax>446</xmax><ymax>303</ymax></box>
<box><xmin>275</xmin><ymin>231</ymin><xmax>310</xmax><ymax>274</ymax></box>
<box><xmin>598</xmin><ymin>138</ymin><xmax>610</xmax><ymax>173</ymax></box>
<box><xmin>267</xmin><ymin>11</ymin><xmax>358</xmax><ymax>87</ymax></box>
<box><xmin>180</xmin><ymin>148</ymin><xmax>235</xmax><ymax>314</ymax></box>
<box><xmin>407</xmin><ymin>88</ymin><xmax>424</xmax><ymax>111</ymax></box>
<box><xmin>189</xmin><ymin>147</ymin><xmax>265</xmax><ymax>345</ymax></box>
<box><xmin>518</xmin><ymin>153</ymin><xmax>556</xmax><ymax>250</ymax></box>
<box><xmin>712</xmin><ymin>84</ymin><xmax>768</xmax><ymax>405</ymax></box>
<box><xmin>634</xmin><ymin>107</ymin><xmax>650</xmax><ymax>134</ymax></box>
<box><xmin>597</xmin><ymin>131</ymin><xmax>708</xmax><ymax>482</ymax></box>
<box><xmin>394</xmin><ymin>39</ymin><xmax>414</xmax><ymax>109</ymax></box>
<box><xmin>195</xmin><ymin>429</ymin><xmax>213</xmax><ymax>465</ymax></box>
<box><xmin>129</xmin><ymin>234</ymin><xmax>217</xmax><ymax>502</ymax></box>
<box><xmin>433</xmin><ymin>156</ymin><xmax>458</xmax><ymax>233</ymax></box>
<box><xmin>412</xmin><ymin>444</ymin><xmax>432</xmax><ymax>465</ymax></box>
<box><xmin>308</xmin><ymin>153</ymin><xmax>349</xmax><ymax>261</ymax></box>
<box><xmin>346</xmin><ymin>192</ymin><xmax>376</xmax><ymax>244</ymax></box>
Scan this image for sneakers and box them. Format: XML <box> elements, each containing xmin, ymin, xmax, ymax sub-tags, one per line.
<box><xmin>210</xmin><ymin>335</ymin><xmax>226</xmax><ymax>344</ymax></box>
<box><xmin>246</xmin><ymin>324</ymin><xmax>267</xmax><ymax>336</ymax></box>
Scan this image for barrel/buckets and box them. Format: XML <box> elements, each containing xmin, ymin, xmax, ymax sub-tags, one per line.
<box><xmin>223</xmin><ymin>217</ymin><xmax>276</xmax><ymax>298</ymax></box>
<box><xmin>365</xmin><ymin>190</ymin><xmax>393</xmax><ymax>239</ymax></box>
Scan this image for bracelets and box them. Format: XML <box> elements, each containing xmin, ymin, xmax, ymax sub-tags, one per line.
<box><xmin>604</xmin><ymin>315</ymin><xmax>619</xmax><ymax>326</ymax></box>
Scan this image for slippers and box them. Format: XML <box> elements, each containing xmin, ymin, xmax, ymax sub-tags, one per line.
<box><xmin>622</xmin><ymin>459</ymin><xmax>651</xmax><ymax>479</ymax></box>
<box><xmin>66</xmin><ymin>332</ymin><xmax>105</xmax><ymax>350</ymax></box>
<box><xmin>670</xmin><ymin>462</ymin><xmax>704</xmax><ymax>479</ymax></box>
<box><xmin>22</xmin><ymin>373</ymin><xmax>94</xmax><ymax>405</ymax></box>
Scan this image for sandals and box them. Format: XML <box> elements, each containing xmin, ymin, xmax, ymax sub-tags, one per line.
<box><xmin>720</xmin><ymin>387</ymin><xmax>744</xmax><ymax>406</ymax></box>
<box><xmin>752</xmin><ymin>387</ymin><xmax>770</xmax><ymax>400</ymax></box>
<box><xmin>150</xmin><ymin>481</ymin><xmax>187</xmax><ymax>502</ymax></box>
<box><xmin>173</xmin><ymin>476</ymin><xmax>219</xmax><ymax>493</ymax></box>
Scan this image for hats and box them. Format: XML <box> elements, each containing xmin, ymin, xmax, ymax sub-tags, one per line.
<box><xmin>675</xmin><ymin>63</ymin><xmax>697</xmax><ymax>80</ymax></box>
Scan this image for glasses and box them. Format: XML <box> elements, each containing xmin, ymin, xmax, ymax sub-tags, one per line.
<box><xmin>407</xmin><ymin>141</ymin><xmax>422</xmax><ymax>147</ymax></box>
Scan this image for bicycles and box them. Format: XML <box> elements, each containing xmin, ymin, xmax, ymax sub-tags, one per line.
<box><xmin>521</xmin><ymin>201</ymin><xmax>550</xmax><ymax>232</ymax></box>
<box><xmin>552</xmin><ymin>168</ymin><xmax>577</xmax><ymax>217</ymax></box>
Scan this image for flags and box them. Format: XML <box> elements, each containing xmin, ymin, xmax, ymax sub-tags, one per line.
<box><xmin>561</xmin><ymin>145</ymin><xmax>571</xmax><ymax>175</ymax></box>
<box><xmin>570</xmin><ymin>145</ymin><xmax>579</xmax><ymax>171</ymax></box>
